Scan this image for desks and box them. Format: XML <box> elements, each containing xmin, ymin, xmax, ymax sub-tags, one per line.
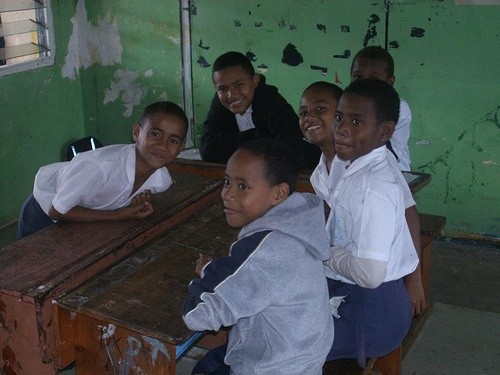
<box><xmin>0</xmin><ymin>147</ymin><xmax>432</xmax><ymax>375</ymax></box>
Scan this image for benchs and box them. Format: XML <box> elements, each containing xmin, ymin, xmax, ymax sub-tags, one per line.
<box><xmin>418</xmin><ymin>213</ymin><xmax>446</xmax><ymax>303</ymax></box>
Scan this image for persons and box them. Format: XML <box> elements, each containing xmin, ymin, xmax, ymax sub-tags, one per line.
<box><xmin>182</xmin><ymin>137</ymin><xmax>335</xmax><ymax>375</ymax></box>
<box><xmin>198</xmin><ymin>51</ymin><xmax>307</xmax><ymax>166</ymax></box>
<box><xmin>297</xmin><ymin>81</ymin><xmax>426</xmax><ymax>316</ymax></box>
<box><xmin>352</xmin><ymin>47</ymin><xmax>410</xmax><ymax>172</ymax></box>
<box><xmin>17</xmin><ymin>102</ymin><xmax>190</xmax><ymax>238</ymax></box>
<box><xmin>322</xmin><ymin>77</ymin><xmax>412</xmax><ymax>362</ymax></box>
